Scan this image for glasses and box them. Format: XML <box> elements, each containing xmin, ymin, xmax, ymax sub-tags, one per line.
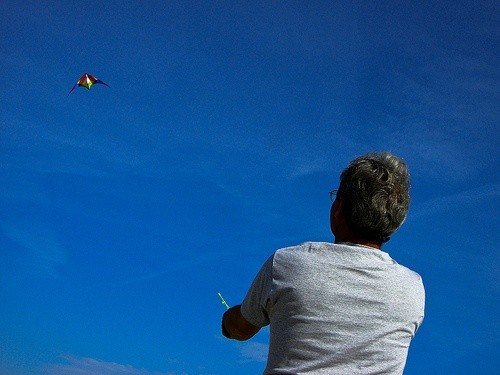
<box><xmin>328</xmin><ymin>188</ymin><xmax>340</xmax><ymax>203</ymax></box>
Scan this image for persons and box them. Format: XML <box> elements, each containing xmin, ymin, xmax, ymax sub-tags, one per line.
<box><xmin>221</xmin><ymin>151</ymin><xmax>426</xmax><ymax>375</ymax></box>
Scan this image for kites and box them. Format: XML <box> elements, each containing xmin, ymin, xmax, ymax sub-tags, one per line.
<box><xmin>68</xmin><ymin>72</ymin><xmax>110</xmax><ymax>95</ymax></box>
